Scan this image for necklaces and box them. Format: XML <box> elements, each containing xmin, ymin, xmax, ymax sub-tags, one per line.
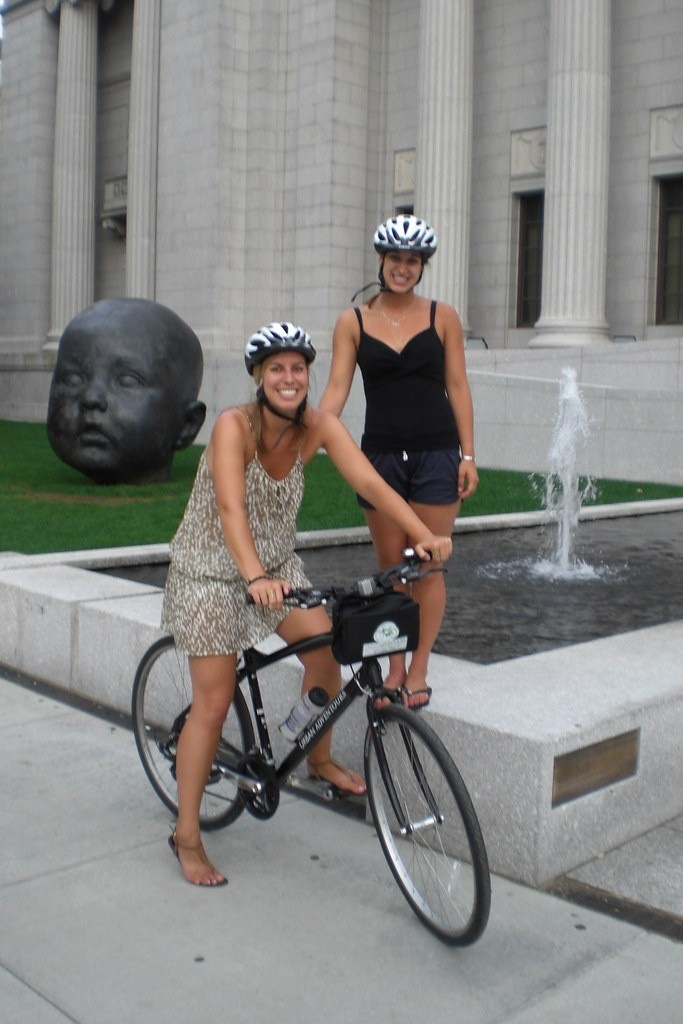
<box><xmin>374</xmin><ymin>293</ymin><xmax>418</xmax><ymax>327</ymax></box>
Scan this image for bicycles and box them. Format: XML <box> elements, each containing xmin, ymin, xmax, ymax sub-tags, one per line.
<box><xmin>131</xmin><ymin>546</ymin><xmax>492</xmax><ymax>949</ymax></box>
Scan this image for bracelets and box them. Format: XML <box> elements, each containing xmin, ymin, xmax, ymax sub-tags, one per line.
<box><xmin>247</xmin><ymin>574</ymin><xmax>272</xmax><ymax>584</ymax></box>
<box><xmin>461</xmin><ymin>453</ymin><xmax>477</xmax><ymax>463</ymax></box>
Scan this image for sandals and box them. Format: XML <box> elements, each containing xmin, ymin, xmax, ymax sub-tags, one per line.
<box><xmin>306</xmin><ymin>759</ymin><xmax>367</xmax><ymax>796</ymax></box>
<box><xmin>167</xmin><ymin>824</ymin><xmax>228</xmax><ymax>887</ymax></box>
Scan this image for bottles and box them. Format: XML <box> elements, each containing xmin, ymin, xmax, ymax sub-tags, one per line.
<box><xmin>278</xmin><ymin>687</ymin><xmax>328</xmax><ymax>743</ymax></box>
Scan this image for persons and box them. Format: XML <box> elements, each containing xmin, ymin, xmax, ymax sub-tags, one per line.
<box><xmin>48</xmin><ymin>296</ymin><xmax>206</xmax><ymax>489</ymax></box>
<box><xmin>161</xmin><ymin>319</ymin><xmax>453</xmax><ymax>895</ymax></box>
<box><xmin>304</xmin><ymin>214</ymin><xmax>480</xmax><ymax>710</ymax></box>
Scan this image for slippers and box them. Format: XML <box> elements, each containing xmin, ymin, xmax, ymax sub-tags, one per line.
<box><xmin>402</xmin><ymin>687</ymin><xmax>432</xmax><ymax>710</ymax></box>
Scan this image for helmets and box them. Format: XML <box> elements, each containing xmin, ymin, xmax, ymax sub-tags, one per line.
<box><xmin>374</xmin><ymin>213</ymin><xmax>438</xmax><ymax>262</ymax></box>
<box><xmin>244</xmin><ymin>321</ymin><xmax>316</xmax><ymax>375</ymax></box>
